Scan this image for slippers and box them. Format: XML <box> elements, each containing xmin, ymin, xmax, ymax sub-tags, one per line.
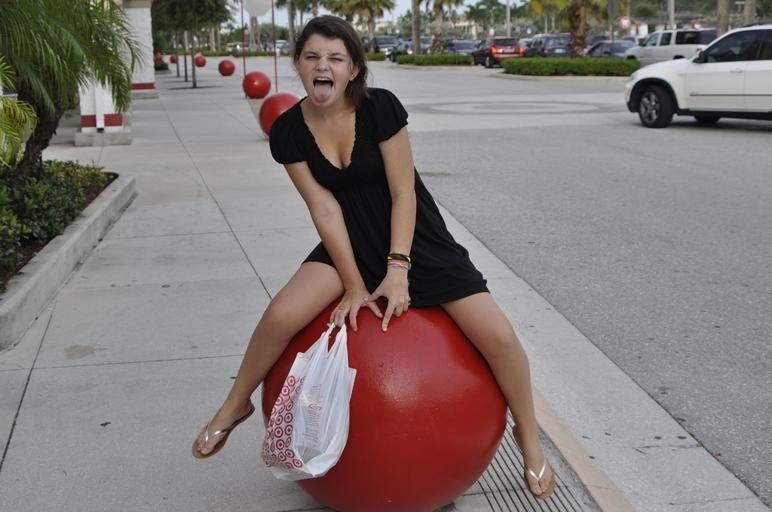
<box><xmin>191</xmin><ymin>399</ymin><xmax>255</xmax><ymax>460</ymax></box>
<box><xmin>513</xmin><ymin>424</ymin><xmax>555</xmax><ymax>501</ymax></box>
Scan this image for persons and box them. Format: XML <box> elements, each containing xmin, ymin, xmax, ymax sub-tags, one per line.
<box><xmin>190</xmin><ymin>14</ymin><xmax>556</xmax><ymax>498</ymax></box>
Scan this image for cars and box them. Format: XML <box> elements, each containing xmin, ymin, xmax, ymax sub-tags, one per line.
<box><xmin>363</xmin><ymin>33</ymin><xmax>571</xmax><ymax>68</ymax></box>
<box><xmin>625</xmin><ymin>24</ymin><xmax>772</xmax><ymax>129</ymax></box>
<box><xmin>583</xmin><ymin>34</ymin><xmax>637</xmax><ymax>57</ymax></box>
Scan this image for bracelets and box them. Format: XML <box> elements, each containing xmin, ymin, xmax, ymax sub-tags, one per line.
<box><xmin>387</xmin><ymin>252</ymin><xmax>412</xmax><ymax>270</ymax></box>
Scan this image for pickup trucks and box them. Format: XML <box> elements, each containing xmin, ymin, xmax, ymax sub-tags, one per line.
<box><xmin>625</xmin><ymin>27</ymin><xmax>716</xmax><ymax>60</ymax></box>
